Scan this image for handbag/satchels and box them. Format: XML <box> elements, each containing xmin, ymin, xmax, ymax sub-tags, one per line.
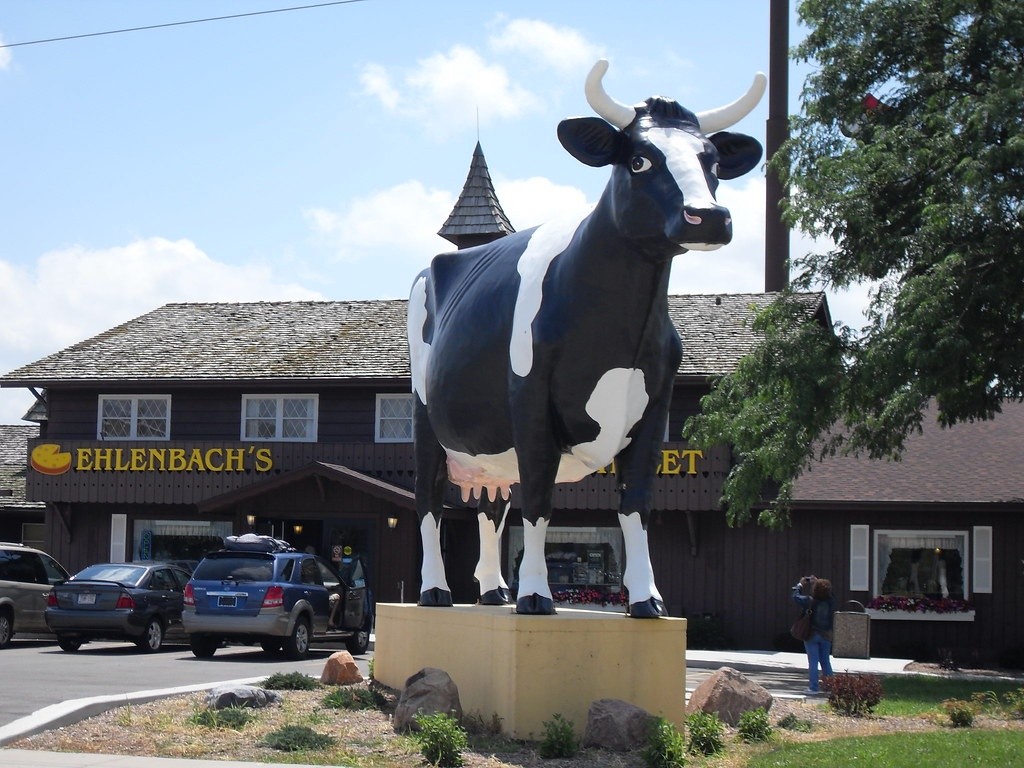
<box><xmin>790</xmin><ymin>596</ymin><xmax>814</xmax><ymax>640</ymax></box>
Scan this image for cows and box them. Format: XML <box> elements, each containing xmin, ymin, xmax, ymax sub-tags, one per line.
<box><xmin>406</xmin><ymin>60</ymin><xmax>767</xmax><ymax>618</ymax></box>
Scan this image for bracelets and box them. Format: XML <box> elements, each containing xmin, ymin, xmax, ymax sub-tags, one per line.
<box><xmin>797</xmin><ymin>583</ymin><xmax>803</xmax><ymax>588</ymax></box>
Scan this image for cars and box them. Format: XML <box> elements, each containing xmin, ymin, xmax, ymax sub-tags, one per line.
<box><xmin>44</xmin><ymin>561</ymin><xmax>195</xmax><ymax>652</ymax></box>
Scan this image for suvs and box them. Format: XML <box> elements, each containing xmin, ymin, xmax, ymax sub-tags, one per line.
<box><xmin>0</xmin><ymin>541</ymin><xmax>73</xmax><ymax>648</ymax></box>
<box><xmin>181</xmin><ymin>533</ymin><xmax>374</xmax><ymax>661</ymax></box>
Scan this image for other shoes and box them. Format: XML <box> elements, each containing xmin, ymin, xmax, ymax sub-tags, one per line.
<box><xmin>327</xmin><ymin>623</ymin><xmax>337</xmax><ymax>628</ymax></box>
<box><xmin>818</xmin><ymin>688</ymin><xmax>832</xmax><ymax>694</ymax></box>
<box><xmin>802</xmin><ymin>688</ymin><xmax>818</xmax><ymax>695</ymax></box>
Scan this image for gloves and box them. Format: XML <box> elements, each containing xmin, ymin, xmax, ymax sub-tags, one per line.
<box><xmin>799</xmin><ymin>575</ymin><xmax>817</xmax><ymax>592</ymax></box>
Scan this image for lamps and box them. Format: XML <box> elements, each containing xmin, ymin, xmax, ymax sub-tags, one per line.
<box><xmin>245</xmin><ymin>509</ymin><xmax>258</xmax><ymax>531</ymax></box>
<box><xmin>387</xmin><ymin>512</ymin><xmax>398</xmax><ymax>533</ymax></box>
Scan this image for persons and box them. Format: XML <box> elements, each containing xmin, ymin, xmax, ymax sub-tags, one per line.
<box><xmin>792</xmin><ymin>574</ymin><xmax>835</xmax><ymax>695</ymax></box>
<box><xmin>328</xmin><ymin>591</ymin><xmax>340</xmax><ymax>628</ymax></box>
